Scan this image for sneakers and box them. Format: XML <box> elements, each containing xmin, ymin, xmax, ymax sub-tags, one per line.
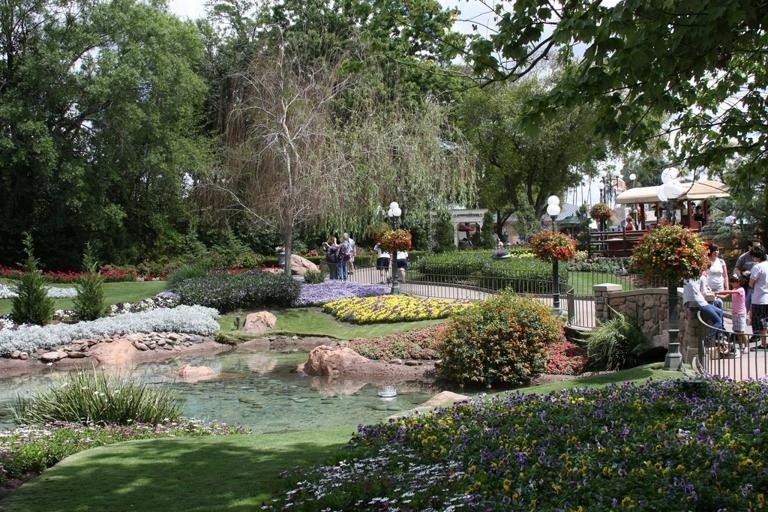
<box><xmin>743</xmin><ymin>332</ymin><xmax>768</xmax><ymax>354</ymax></box>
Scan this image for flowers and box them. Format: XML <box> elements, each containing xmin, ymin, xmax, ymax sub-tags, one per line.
<box><xmin>622</xmin><ymin>219</ymin><xmax>712</xmax><ymax>291</ymax></box>
<box><xmin>376</xmin><ymin>229</ymin><xmax>413</xmax><ymax>253</ymax></box>
<box><xmin>528</xmin><ymin>230</ymin><xmax>579</xmax><ymax>264</ymax></box>
<box><xmin>589</xmin><ymin>202</ymin><xmax>613</xmax><ymax>221</ymax></box>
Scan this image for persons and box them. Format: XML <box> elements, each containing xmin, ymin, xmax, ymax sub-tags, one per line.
<box><xmin>374</xmin><ymin>242</ymin><xmax>391</xmax><ymax>284</ymax></box>
<box><xmin>565</xmin><ymin>226</ymin><xmax>572</xmax><ymax>237</ymax></box>
<box><xmin>397</xmin><ymin>249</ymin><xmax>409</xmax><ymax>285</ymax></box>
<box><xmin>321</xmin><ymin>232</ymin><xmax>356</xmax><ymax>281</ymax></box>
<box><xmin>493</xmin><ymin>232</ymin><xmax>523</xmax><ymax>250</ymax></box>
<box><xmin>723</xmin><ymin>210</ymin><xmax>736</xmax><ymax>228</ymax></box>
<box><xmin>681</xmin><ymin>238</ymin><xmax>768</xmax><ymax>360</ymax></box>
<box><xmin>694</xmin><ymin>205</ymin><xmax>706</xmax><ymax>227</ymax></box>
<box><xmin>625</xmin><ymin>214</ymin><xmax>641</xmax><ymax>230</ymax></box>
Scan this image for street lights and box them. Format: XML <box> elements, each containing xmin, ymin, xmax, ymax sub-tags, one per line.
<box><xmin>659</xmin><ymin>168</ymin><xmax>681</xmax><ymax>344</ymax></box>
<box><xmin>546</xmin><ymin>196</ymin><xmax>560</xmax><ymax>309</ymax></box>
<box><xmin>629</xmin><ymin>172</ymin><xmax>639</xmax><ymax>231</ymax></box>
<box><xmin>388</xmin><ymin>201</ymin><xmax>401</xmax><ymax>294</ymax></box>
